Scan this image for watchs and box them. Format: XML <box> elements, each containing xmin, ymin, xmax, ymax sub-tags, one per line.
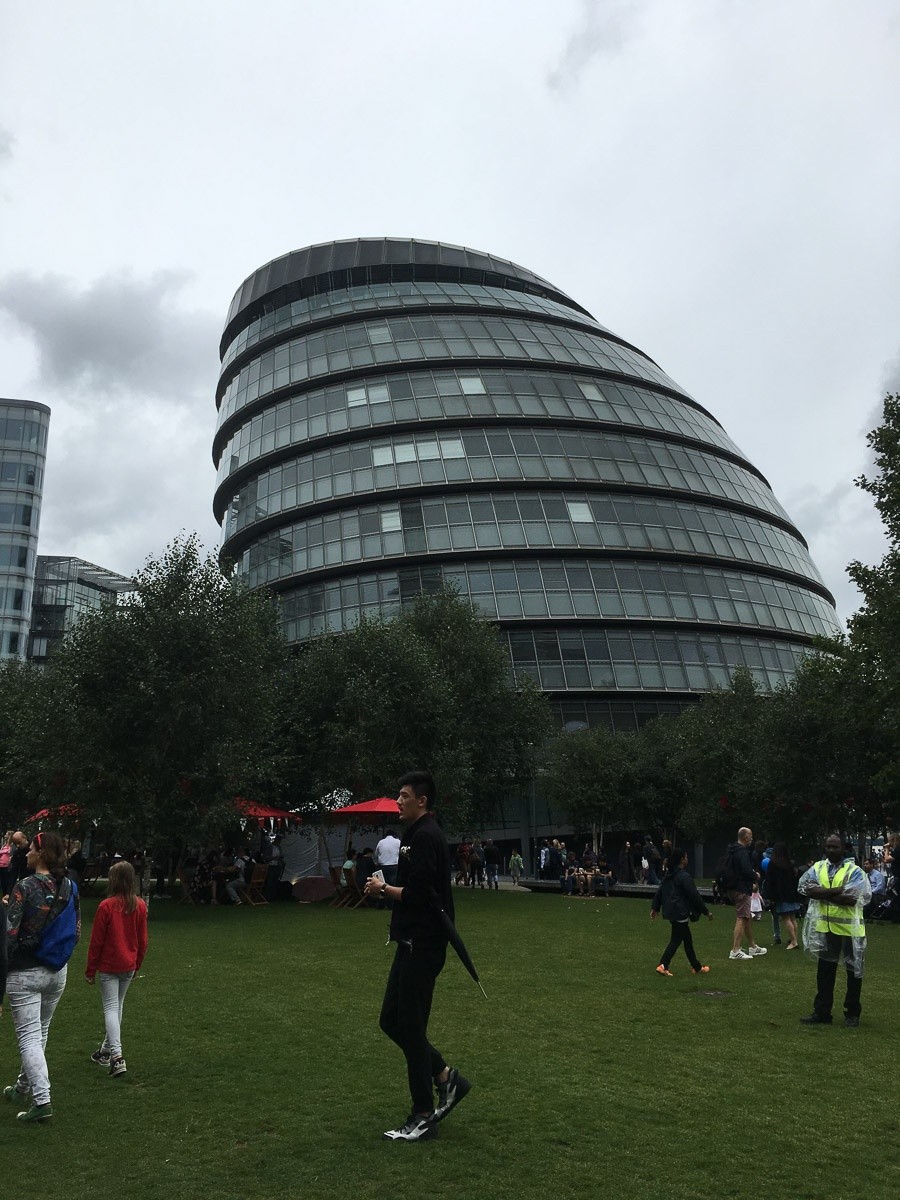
<box><xmin>380</xmin><ymin>883</ymin><xmax>389</xmax><ymax>898</ymax></box>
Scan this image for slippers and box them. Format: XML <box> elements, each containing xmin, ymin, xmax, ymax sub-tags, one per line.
<box><xmin>787</xmin><ymin>943</ymin><xmax>799</xmax><ymax>950</ymax></box>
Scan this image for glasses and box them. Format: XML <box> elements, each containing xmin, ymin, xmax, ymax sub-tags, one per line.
<box><xmin>34</xmin><ymin>831</ymin><xmax>46</xmax><ymax>851</ymax></box>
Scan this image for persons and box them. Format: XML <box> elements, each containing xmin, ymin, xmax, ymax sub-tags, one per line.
<box><xmin>364</xmin><ymin>769</ymin><xmax>472</xmax><ymax>1142</ymax></box>
<box><xmin>539</xmin><ymin>838</ymin><xmax>673</xmax><ymax>899</ymax></box>
<box><xmin>455</xmin><ymin>838</ymin><xmax>500</xmax><ymax>890</ymax></box>
<box><xmin>649</xmin><ymin>848</ymin><xmax>714</xmax><ymax>977</ymax></box>
<box><xmin>509</xmin><ymin>848</ymin><xmax>525</xmax><ymax>886</ymax></box>
<box><xmin>190</xmin><ymin>850</ymin><xmax>267</xmax><ymax>907</ymax></box>
<box><xmin>798</xmin><ymin>836</ymin><xmax>873</xmax><ymax>1028</ymax></box>
<box><xmin>84</xmin><ymin>861</ymin><xmax>149</xmax><ymax>1078</ymax></box>
<box><xmin>713</xmin><ymin>827</ymin><xmax>801</xmax><ymax>960</ymax></box>
<box><xmin>863</xmin><ymin>833</ymin><xmax>900</xmax><ymax>922</ymax></box>
<box><xmin>0</xmin><ymin>831</ymin><xmax>84</xmax><ymax>1122</ymax></box>
<box><xmin>340</xmin><ymin>830</ymin><xmax>400</xmax><ymax>907</ymax></box>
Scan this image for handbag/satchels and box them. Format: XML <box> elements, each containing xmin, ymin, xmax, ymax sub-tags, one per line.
<box><xmin>36</xmin><ymin>879</ymin><xmax>78</xmax><ymax>971</ymax></box>
<box><xmin>750</xmin><ymin>886</ymin><xmax>765</xmax><ymax>912</ymax></box>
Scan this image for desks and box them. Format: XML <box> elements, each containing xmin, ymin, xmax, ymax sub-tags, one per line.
<box><xmin>213</xmin><ymin>870</ymin><xmax>238</xmax><ymax>901</ymax></box>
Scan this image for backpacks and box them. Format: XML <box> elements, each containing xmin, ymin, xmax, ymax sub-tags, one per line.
<box><xmin>238</xmin><ymin>856</ymin><xmax>256</xmax><ymax>884</ymax></box>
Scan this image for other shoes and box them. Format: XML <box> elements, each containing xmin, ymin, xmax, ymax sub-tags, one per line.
<box><xmin>453</xmin><ymin>880</ymin><xmax>499</xmax><ymax>890</ymax></box>
<box><xmin>560</xmin><ymin>891</ymin><xmax>609</xmax><ymax>897</ymax></box>
<box><xmin>153</xmin><ymin>893</ymin><xmax>173</xmax><ymax>900</ymax></box>
<box><xmin>210</xmin><ymin>899</ymin><xmax>221</xmax><ymax>905</ymax></box>
<box><xmin>234</xmin><ymin>900</ymin><xmax>242</xmax><ymax>906</ymax></box>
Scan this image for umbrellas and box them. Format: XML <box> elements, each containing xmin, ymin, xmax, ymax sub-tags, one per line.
<box><xmin>330</xmin><ymin>797</ymin><xmax>401</xmax><ymax>860</ymax></box>
<box><xmin>401</xmin><ymin>857</ymin><xmax>489</xmax><ymax>1002</ymax></box>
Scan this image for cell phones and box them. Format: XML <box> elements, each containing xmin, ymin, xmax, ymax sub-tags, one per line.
<box><xmin>372</xmin><ymin>869</ymin><xmax>386</xmax><ymax>891</ymax></box>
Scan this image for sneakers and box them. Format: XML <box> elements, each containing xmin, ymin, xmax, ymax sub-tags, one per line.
<box><xmin>800</xmin><ymin>1010</ymin><xmax>833</xmax><ymax>1026</ymax></box>
<box><xmin>16</xmin><ymin>1102</ymin><xmax>54</xmax><ymax>1122</ymax></box>
<box><xmin>749</xmin><ymin>946</ymin><xmax>767</xmax><ymax>955</ymax></box>
<box><xmin>434</xmin><ymin>1068</ymin><xmax>472</xmax><ymax>1122</ymax></box>
<box><xmin>844</xmin><ymin>1013</ymin><xmax>860</xmax><ymax>1027</ymax></box>
<box><xmin>382</xmin><ymin>1112</ymin><xmax>437</xmax><ymax>1141</ymax></box>
<box><xmin>691</xmin><ymin>966</ymin><xmax>710</xmax><ymax>973</ymax></box>
<box><xmin>5</xmin><ymin>1086</ymin><xmax>29</xmax><ymax>1105</ymax></box>
<box><xmin>91</xmin><ymin>1050</ymin><xmax>110</xmax><ymax>1066</ymax></box>
<box><xmin>108</xmin><ymin>1059</ymin><xmax>127</xmax><ymax>1078</ymax></box>
<box><xmin>656</xmin><ymin>966</ymin><xmax>673</xmax><ymax>977</ymax></box>
<box><xmin>729</xmin><ymin>948</ymin><xmax>753</xmax><ymax>960</ymax></box>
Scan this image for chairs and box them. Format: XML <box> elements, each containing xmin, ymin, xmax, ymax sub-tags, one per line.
<box><xmin>238</xmin><ymin>863</ymin><xmax>289</xmax><ymax>906</ymax></box>
<box><xmin>78</xmin><ymin>863</ymin><xmax>103</xmax><ymax>897</ymax></box>
<box><xmin>176</xmin><ymin>858</ymin><xmax>202</xmax><ymax>904</ymax></box>
<box><xmin>329</xmin><ymin>865</ymin><xmax>370</xmax><ymax>910</ymax></box>
